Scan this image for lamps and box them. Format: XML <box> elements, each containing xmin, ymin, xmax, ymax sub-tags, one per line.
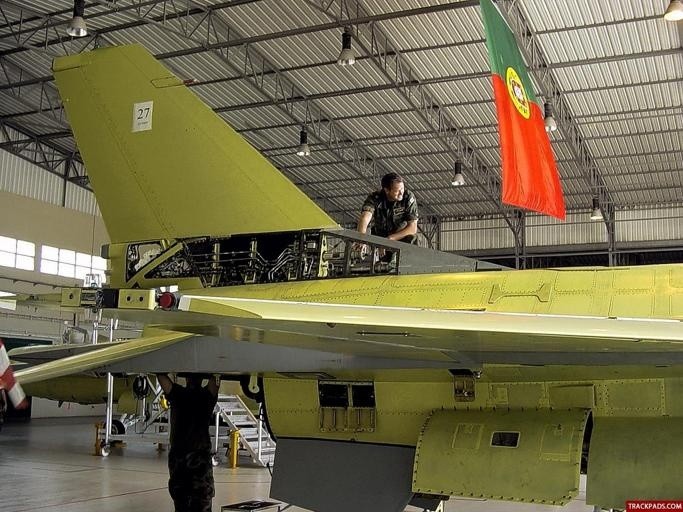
<box><xmin>540</xmin><ymin>95</ymin><xmax>556</xmax><ymax>133</ymax></box>
<box><xmin>294</xmin><ymin>121</ymin><xmax>313</xmax><ymax>157</ymax></box>
<box><xmin>590</xmin><ymin>187</ymin><xmax>605</xmax><ymax>224</ymax></box>
<box><xmin>449</xmin><ymin>155</ymin><xmax>465</xmax><ymax>187</ymax></box>
<box><xmin>664</xmin><ymin>2</ymin><xmax>683</xmax><ymax>22</ymax></box>
<box><xmin>62</xmin><ymin>2</ymin><xmax>90</xmax><ymax>37</ymax></box>
<box><xmin>337</xmin><ymin>29</ymin><xmax>354</xmax><ymax>68</ymax></box>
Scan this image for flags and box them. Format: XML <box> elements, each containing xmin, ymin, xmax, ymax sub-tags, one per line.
<box><xmin>477</xmin><ymin>1</ymin><xmax>566</xmax><ymax>223</ymax></box>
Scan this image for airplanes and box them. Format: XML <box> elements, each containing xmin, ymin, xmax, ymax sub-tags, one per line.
<box><xmin>5</xmin><ymin>354</ymin><xmax>262</xmax><ymax>446</ymax></box>
<box><xmin>5</xmin><ymin>45</ymin><xmax>683</xmax><ymax>511</ymax></box>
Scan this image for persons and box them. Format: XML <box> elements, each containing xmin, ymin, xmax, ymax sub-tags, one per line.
<box><xmin>154</xmin><ymin>371</ymin><xmax>218</xmax><ymax>512</ymax></box>
<box><xmin>348</xmin><ymin>171</ymin><xmax>419</xmax><ymax>258</ymax></box>
